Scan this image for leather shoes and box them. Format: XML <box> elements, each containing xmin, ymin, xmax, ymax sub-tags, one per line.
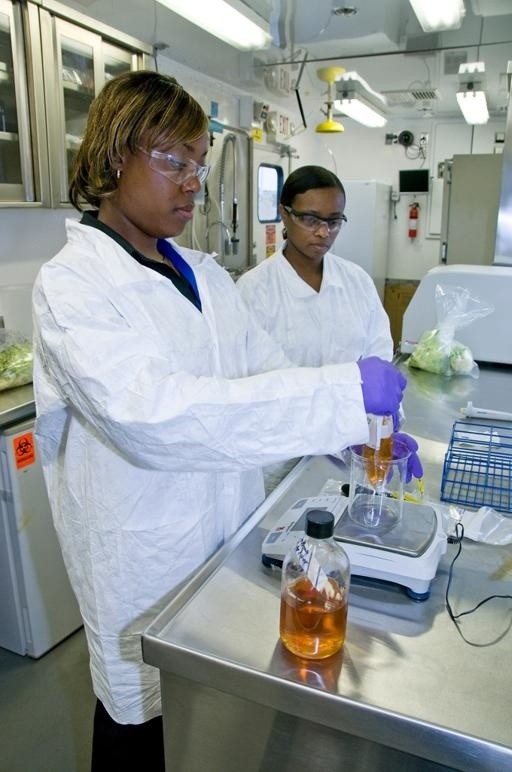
<box><xmin>351</xmin><ymin>431</ymin><xmax>423</xmax><ymax>484</ymax></box>
<box><xmin>355</xmin><ymin>357</ymin><xmax>406</xmax><ymax>415</ymax></box>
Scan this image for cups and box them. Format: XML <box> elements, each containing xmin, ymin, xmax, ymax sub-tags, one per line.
<box><xmin>279</xmin><ymin>203</ymin><xmax>348</xmax><ymax>235</ymax></box>
<box><xmin>133</xmin><ymin>145</ymin><xmax>211</xmax><ymax>184</ymax></box>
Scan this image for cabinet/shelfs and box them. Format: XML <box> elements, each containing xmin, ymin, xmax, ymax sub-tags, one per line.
<box><xmin>279</xmin><ymin>509</ymin><xmax>350</xmax><ymax>658</ymax></box>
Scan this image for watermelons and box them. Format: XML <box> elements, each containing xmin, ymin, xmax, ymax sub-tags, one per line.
<box><xmin>261</xmin><ymin>494</ymin><xmax>456</xmax><ymax>598</ymax></box>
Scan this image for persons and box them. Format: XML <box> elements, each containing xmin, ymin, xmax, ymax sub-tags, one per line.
<box><xmin>33</xmin><ymin>71</ymin><xmax>422</xmax><ymax>772</ymax></box>
<box><xmin>233</xmin><ymin>166</ymin><xmax>395</xmax><ymax>496</ymax></box>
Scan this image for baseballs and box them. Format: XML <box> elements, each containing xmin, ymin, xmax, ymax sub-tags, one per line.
<box><xmin>408</xmin><ymin>202</ymin><xmax>421</xmax><ymax>237</ymax></box>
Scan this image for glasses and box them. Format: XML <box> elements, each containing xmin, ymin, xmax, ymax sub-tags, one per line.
<box><xmin>315</xmin><ymin>100</ymin><xmax>343</xmax><ymax>132</ymax></box>
<box><xmin>315</xmin><ymin>66</ymin><xmax>345</xmax><ymax>101</ymax></box>
<box><xmin>158</xmin><ymin>1</ymin><xmax>272</xmax><ymax>54</ymax></box>
<box><xmin>333</xmin><ymin>91</ymin><xmax>388</xmax><ymax>129</ymax></box>
<box><xmin>334</xmin><ymin>71</ymin><xmax>388</xmax><ymax>114</ymax></box>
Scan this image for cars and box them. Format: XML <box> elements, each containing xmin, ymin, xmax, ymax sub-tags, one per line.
<box><xmin>347</xmin><ymin>439</ymin><xmax>412</xmax><ymax>531</ymax></box>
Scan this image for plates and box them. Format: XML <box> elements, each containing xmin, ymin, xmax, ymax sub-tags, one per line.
<box><xmin>0</xmin><ymin>0</ymin><xmax>157</xmax><ymax>208</ymax></box>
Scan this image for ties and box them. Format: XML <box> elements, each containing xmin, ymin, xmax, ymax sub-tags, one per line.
<box><xmin>398</xmin><ymin>168</ymin><xmax>430</xmax><ymax>195</ymax></box>
